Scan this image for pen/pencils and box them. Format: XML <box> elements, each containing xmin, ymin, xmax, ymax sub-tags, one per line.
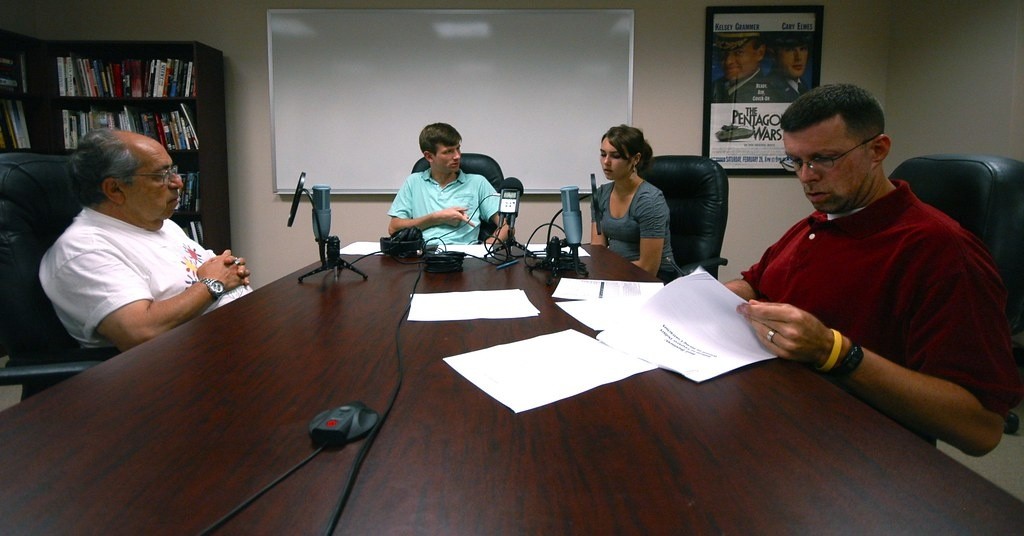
<box><xmin>496</xmin><ymin>260</ymin><xmax>521</xmax><ymax>269</ymax></box>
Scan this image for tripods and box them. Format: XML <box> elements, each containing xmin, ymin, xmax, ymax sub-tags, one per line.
<box><xmin>484</xmin><ymin>228</ymin><xmax>537</xmax><ymax>258</ymax></box>
<box><xmin>529</xmin><ymin>235</ymin><xmax>589</xmax><ymax>285</ymax></box>
<box><xmin>298</xmin><ymin>236</ymin><xmax>368</xmax><ymax>282</ymax></box>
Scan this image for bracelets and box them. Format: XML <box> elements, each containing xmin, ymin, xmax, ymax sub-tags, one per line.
<box><xmin>816</xmin><ymin>329</ymin><xmax>842</xmax><ymax>373</ymax></box>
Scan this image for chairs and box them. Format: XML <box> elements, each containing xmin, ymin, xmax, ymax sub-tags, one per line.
<box><xmin>411</xmin><ymin>153</ymin><xmax>506</xmax><ymax>244</ymax></box>
<box><xmin>637</xmin><ymin>154</ymin><xmax>729</xmax><ymax>287</ymax></box>
<box><xmin>886</xmin><ymin>153</ymin><xmax>1024</xmax><ymax>434</ymax></box>
<box><xmin>0</xmin><ymin>149</ymin><xmax>121</xmax><ymax>401</ymax></box>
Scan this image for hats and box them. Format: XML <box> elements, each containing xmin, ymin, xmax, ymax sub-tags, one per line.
<box><xmin>715</xmin><ymin>32</ymin><xmax>760</xmax><ymax>51</ymax></box>
<box><xmin>766</xmin><ymin>32</ymin><xmax>810</xmax><ymax>46</ymax></box>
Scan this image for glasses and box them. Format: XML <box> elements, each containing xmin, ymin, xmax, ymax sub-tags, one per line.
<box><xmin>97</xmin><ymin>165</ymin><xmax>178</xmax><ymax>185</ymax></box>
<box><xmin>780</xmin><ymin>132</ymin><xmax>882</xmax><ymax>171</ymax></box>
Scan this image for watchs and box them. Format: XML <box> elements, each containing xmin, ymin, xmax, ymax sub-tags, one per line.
<box><xmin>832</xmin><ymin>340</ymin><xmax>864</xmax><ymax>378</ymax></box>
<box><xmin>200</xmin><ymin>277</ymin><xmax>226</xmax><ymax>299</ymax></box>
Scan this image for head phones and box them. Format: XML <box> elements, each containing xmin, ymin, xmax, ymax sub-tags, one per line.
<box><xmin>379</xmin><ymin>226</ymin><xmax>427</xmax><ymax>256</ymax></box>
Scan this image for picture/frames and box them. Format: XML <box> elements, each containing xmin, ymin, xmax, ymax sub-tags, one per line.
<box><xmin>701</xmin><ymin>4</ymin><xmax>824</xmax><ymax>178</ymax></box>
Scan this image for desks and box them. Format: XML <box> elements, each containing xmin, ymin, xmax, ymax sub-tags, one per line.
<box><xmin>1</xmin><ymin>242</ymin><xmax>1024</xmax><ymax>536</ymax></box>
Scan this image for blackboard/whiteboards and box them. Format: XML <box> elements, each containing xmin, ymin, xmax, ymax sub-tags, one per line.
<box><xmin>267</xmin><ymin>8</ymin><xmax>636</xmax><ymax>194</ymax></box>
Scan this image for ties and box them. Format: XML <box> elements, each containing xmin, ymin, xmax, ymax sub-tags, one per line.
<box><xmin>798</xmin><ymin>83</ymin><xmax>806</xmax><ymax>95</ymax></box>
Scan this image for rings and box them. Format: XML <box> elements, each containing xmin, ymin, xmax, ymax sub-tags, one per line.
<box><xmin>767</xmin><ymin>330</ymin><xmax>775</xmax><ymax>342</ymax></box>
<box><xmin>233</xmin><ymin>260</ymin><xmax>241</xmax><ymax>265</ymax></box>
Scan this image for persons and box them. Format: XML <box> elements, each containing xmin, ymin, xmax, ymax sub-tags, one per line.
<box><xmin>722</xmin><ymin>84</ymin><xmax>1024</xmax><ymax>458</ymax></box>
<box><xmin>712</xmin><ymin>31</ymin><xmax>778</xmax><ymax>103</ymax></box>
<box><xmin>39</xmin><ymin>128</ymin><xmax>257</xmax><ymax>353</ymax></box>
<box><xmin>767</xmin><ymin>32</ymin><xmax>811</xmax><ymax>103</ymax></box>
<box><xmin>387</xmin><ymin>123</ymin><xmax>509</xmax><ymax>246</ymax></box>
<box><xmin>589</xmin><ymin>124</ymin><xmax>675</xmax><ymax>281</ymax></box>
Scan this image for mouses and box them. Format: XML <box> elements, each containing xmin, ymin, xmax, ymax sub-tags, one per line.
<box><xmin>308</xmin><ymin>402</ymin><xmax>380</xmax><ymax>449</ymax></box>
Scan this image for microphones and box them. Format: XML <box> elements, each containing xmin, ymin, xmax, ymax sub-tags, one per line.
<box><xmin>312</xmin><ymin>185</ymin><xmax>332</xmax><ymax>263</ymax></box>
<box><xmin>499</xmin><ymin>177</ymin><xmax>524</xmax><ymax>229</ymax></box>
<box><xmin>561</xmin><ymin>186</ymin><xmax>582</xmax><ymax>272</ymax></box>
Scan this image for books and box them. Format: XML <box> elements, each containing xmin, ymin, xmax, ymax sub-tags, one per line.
<box><xmin>166</xmin><ymin>172</ymin><xmax>201</xmax><ymax>212</ymax></box>
<box><xmin>62</xmin><ymin>103</ymin><xmax>200</xmax><ymax>151</ymax></box>
<box><xmin>183</xmin><ymin>221</ymin><xmax>205</xmax><ymax>248</ymax></box>
<box><xmin>0</xmin><ymin>99</ymin><xmax>31</xmax><ymax>149</ymax></box>
<box><xmin>0</xmin><ymin>52</ymin><xmax>28</xmax><ymax>93</ymax></box>
<box><xmin>57</xmin><ymin>52</ymin><xmax>197</xmax><ymax>98</ymax></box>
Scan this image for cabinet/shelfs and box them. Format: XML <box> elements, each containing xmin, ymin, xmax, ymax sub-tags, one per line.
<box><xmin>0</xmin><ymin>29</ymin><xmax>232</xmax><ymax>258</ymax></box>
